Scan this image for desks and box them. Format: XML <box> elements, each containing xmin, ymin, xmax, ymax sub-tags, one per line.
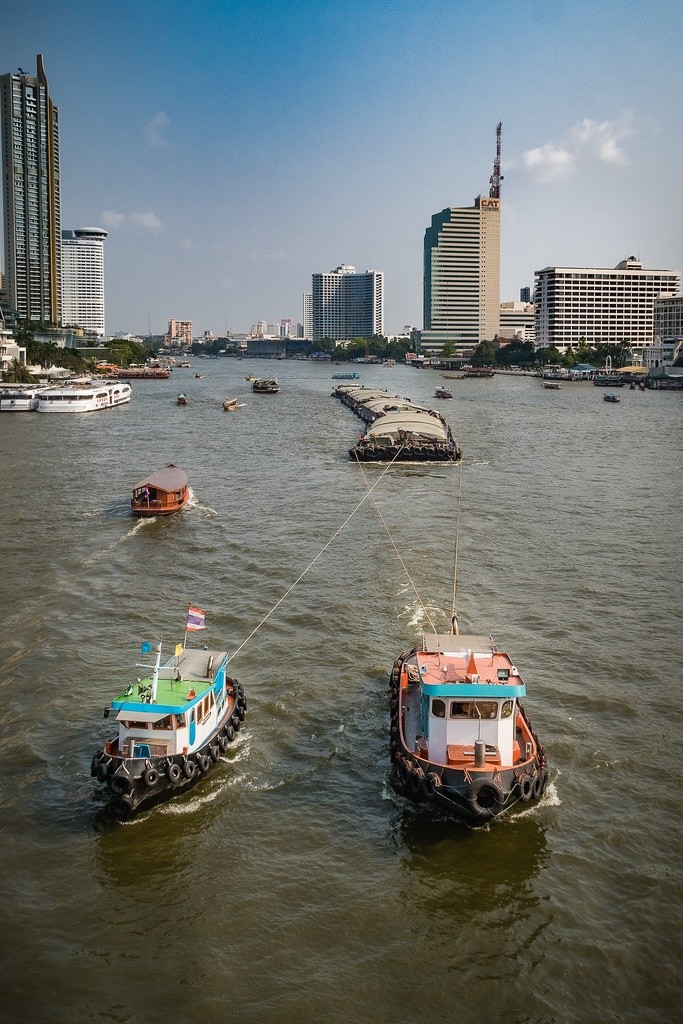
<box><xmin>447</xmin><ymin>745</ymin><xmax>501</xmax><ymax>766</ymax></box>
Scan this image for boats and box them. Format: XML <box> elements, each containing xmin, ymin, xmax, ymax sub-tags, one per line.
<box><xmin>440</xmin><ymin>373</ymin><xmax>465</xmax><ymax>379</ymax></box>
<box><xmin>177</xmin><ymin>361</ymin><xmax>191</xmax><ymax>368</ymax></box>
<box><xmin>252</xmin><ymin>379</ymin><xmax>280</xmax><ymax>394</ymax></box>
<box><xmin>331</xmin><ymin>371</ymin><xmax>360</xmax><ymax>380</ymax></box>
<box><xmin>223</xmin><ymin>398</ymin><xmax>238</xmax><ymax>411</ymax></box>
<box><xmin>603</xmin><ymin>393</ymin><xmax>621</xmax><ymax>402</ymax></box>
<box><xmin>89</xmin><ymin>444</ymin><xmax>406</xmax><ymax>821</ymax></box>
<box><xmin>592</xmin><ymin>374</ymin><xmax>625</xmax><ymax>387</ymax></box>
<box><xmin>351</xmin><ymin>445</ymin><xmax>552</xmax><ymax>827</ymax></box>
<box><xmin>466</xmin><ymin>367</ymin><xmax>494</xmax><ymax>377</ymax></box>
<box><xmin>245</xmin><ymin>375</ymin><xmax>261</xmax><ymax>382</ymax></box>
<box><xmin>435</xmin><ymin>385</ymin><xmax>453</xmax><ymax>398</ymax></box>
<box><xmin>0</xmin><ymin>380</ymin><xmax>135</xmax><ymax>413</ymax></box>
<box><xmin>544</xmin><ymin>381</ymin><xmax>563</xmax><ymax>389</ymax></box>
<box><xmin>176</xmin><ymin>393</ymin><xmax>188</xmax><ymax>406</ymax></box>
<box><xmin>129</xmin><ymin>463</ymin><xmax>189</xmax><ymax>518</ymax></box>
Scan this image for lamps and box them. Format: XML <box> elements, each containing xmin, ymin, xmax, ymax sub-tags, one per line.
<box><xmin>496</xmin><ymin>669</ymin><xmax>510</xmax><ymax>681</ymax></box>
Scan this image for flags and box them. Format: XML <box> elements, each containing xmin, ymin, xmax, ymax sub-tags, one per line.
<box><xmin>142</xmin><ymin>642</ymin><xmax>152</xmax><ymax>654</ymax></box>
<box><xmin>145</xmin><ymin>488</ymin><xmax>149</xmax><ymax>498</ymax></box>
<box><xmin>186</xmin><ymin>606</ymin><xmax>206</xmax><ymax>631</ymax></box>
<box><xmin>176</xmin><ymin>644</ymin><xmax>182</xmax><ymax>656</ymax></box>
